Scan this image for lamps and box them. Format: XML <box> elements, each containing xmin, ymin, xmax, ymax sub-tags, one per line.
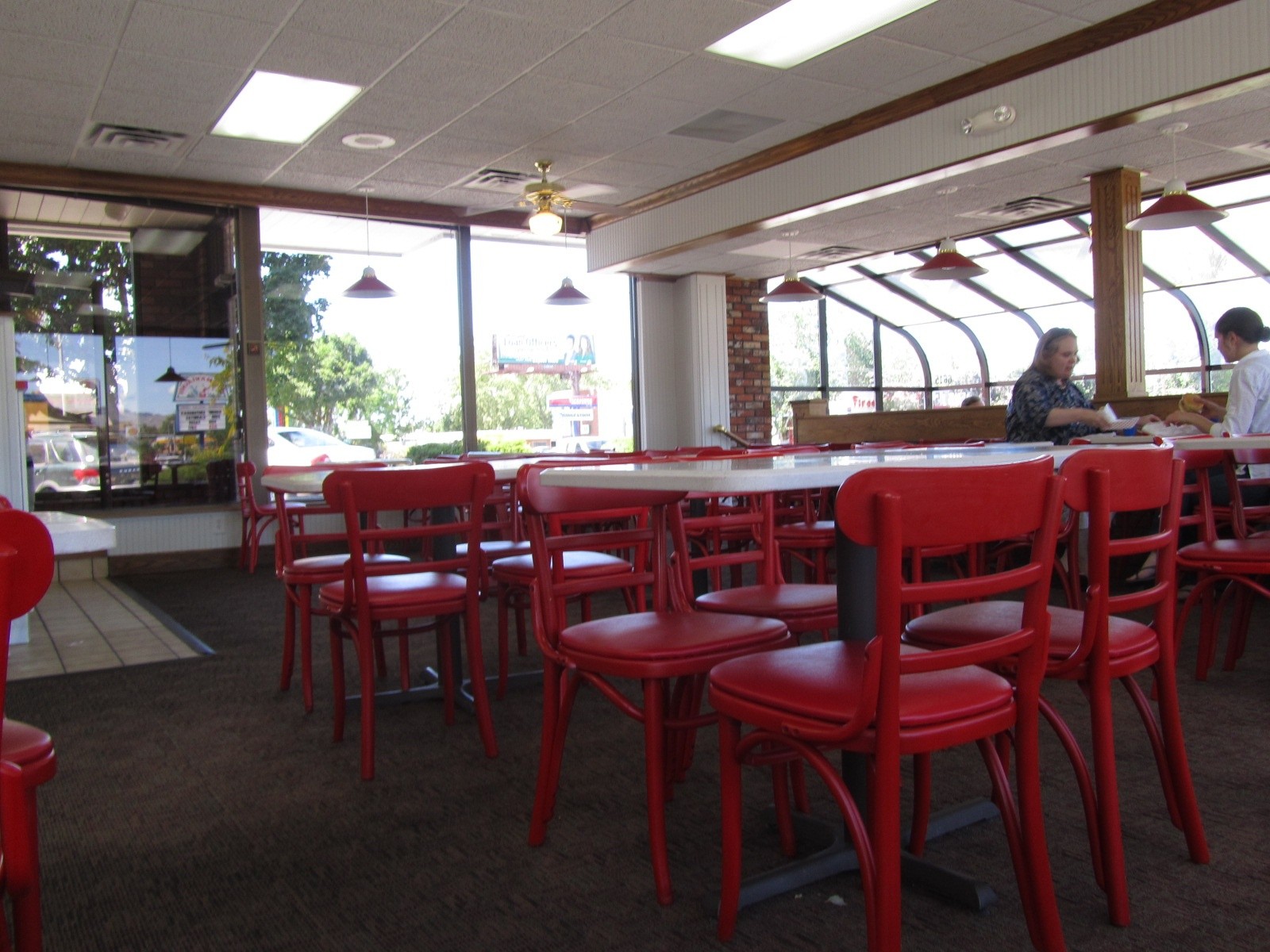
<box><xmin>152</xmin><ymin>309</ymin><xmax>189</xmax><ymax>386</ymax></box>
<box><xmin>757</xmin><ymin>229</ymin><xmax>826</xmax><ymax>305</ymax></box>
<box><xmin>908</xmin><ymin>182</ymin><xmax>989</xmax><ymax>282</ymax></box>
<box><xmin>515</xmin><ymin>158</ymin><xmax>575</xmax><ymax>237</ymax></box>
<box><xmin>341</xmin><ymin>184</ymin><xmax>397</xmax><ymax>299</ymax></box>
<box><xmin>545</xmin><ymin>198</ymin><xmax>590</xmax><ymax>308</ymax></box>
<box><xmin>1124</xmin><ymin>120</ymin><xmax>1231</xmax><ymax>234</ymax></box>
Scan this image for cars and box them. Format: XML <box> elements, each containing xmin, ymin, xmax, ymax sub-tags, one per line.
<box><xmin>266</xmin><ymin>426</ymin><xmax>361</xmax><ymax>471</ymax></box>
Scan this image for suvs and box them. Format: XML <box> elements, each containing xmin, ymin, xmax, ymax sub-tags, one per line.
<box><xmin>26</xmin><ymin>431</ymin><xmax>141</xmax><ymax>496</ymax></box>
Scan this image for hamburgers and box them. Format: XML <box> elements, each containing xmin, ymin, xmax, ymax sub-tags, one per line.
<box><xmin>1179</xmin><ymin>394</ymin><xmax>1204</xmax><ymax>415</ymax></box>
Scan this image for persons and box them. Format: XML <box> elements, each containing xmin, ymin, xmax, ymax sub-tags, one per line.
<box><xmin>959</xmin><ymin>396</ymin><xmax>985</xmax><ymax>407</ymax></box>
<box><xmin>1003</xmin><ymin>328</ymin><xmax>1162</xmax><ymax>591</ymax></box>
<box><xmin>1121</xmin><ymin>306</ymin><xmax>1270</xmax><ymax>584</ymax></box>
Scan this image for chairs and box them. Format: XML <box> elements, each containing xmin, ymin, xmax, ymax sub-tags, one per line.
<box><xmin>0</xmin><ymin>490</ymin><xmax>63</xmax><ymax>952</ymax></box>
<box><xmin>229</xmin><ymin>428</ymin><xmax>1270</xmax><ymax>952</ymax></box>
